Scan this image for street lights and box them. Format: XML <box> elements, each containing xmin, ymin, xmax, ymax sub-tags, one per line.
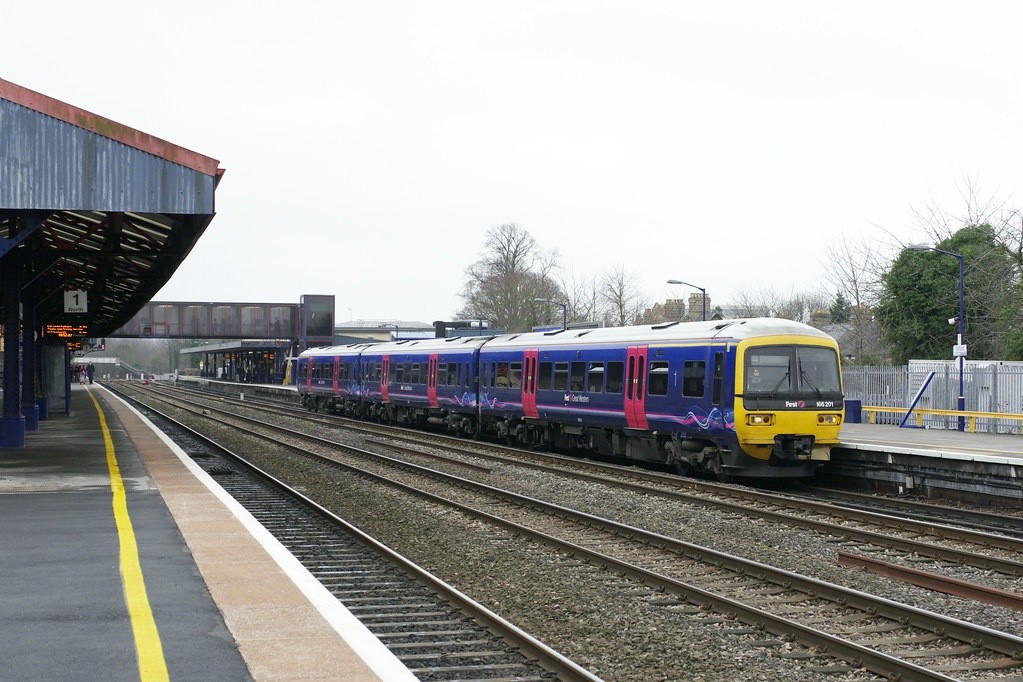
<box><xmin>667</xmin><ymin>280</ymin><xmax>705</xmax><ymax>322</ymax></box>
<box><xmin>378</xmin><ymin>324</ymin><xmax>398</xmax><ymax>341</ymax></box>
<box><xmin>908</xmin><ymin>244</ymin><xmax>965</xmax><ymax>432</ymax></box>
<box><xmin>534</xmin><ymin>298</ymin><xmax>566</xmax><ymax>330</ymax></box>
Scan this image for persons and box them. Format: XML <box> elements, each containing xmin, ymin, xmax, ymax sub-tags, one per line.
<box><xmin>274</xmin><ymin>317</ymin><xmax>282</xmax><ymax>336</ymax></box>
<box><xmin>191</xmin><ymin>315</ymin><xmax>235</xmax><ymax>336</ymax></box>
<box><xmin>69</xmin><ymin>361</ymin><xmax>95</xmax><ymax>384</ymax></box>
<box><xmin>118</xmin><ymin>317</ymin><xmax>136</xmax><ymax>335</ymax></box>
<box><xmin>236</xmin><ymin>365</ymin><xmax>251</xmax><ymax>382</ymax></box>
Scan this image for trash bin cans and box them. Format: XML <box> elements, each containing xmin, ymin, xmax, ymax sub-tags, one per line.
<box><xmin>221</xmin><ymin>374</ymin><xmax>227</xmax><ymax>381</ymax></box>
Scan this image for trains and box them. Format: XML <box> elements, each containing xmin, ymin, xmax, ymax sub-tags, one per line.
<box><xmin>296</xmin><ymin>317</ymin><xmax>846</xmax><ymax>482</ymax></box>
